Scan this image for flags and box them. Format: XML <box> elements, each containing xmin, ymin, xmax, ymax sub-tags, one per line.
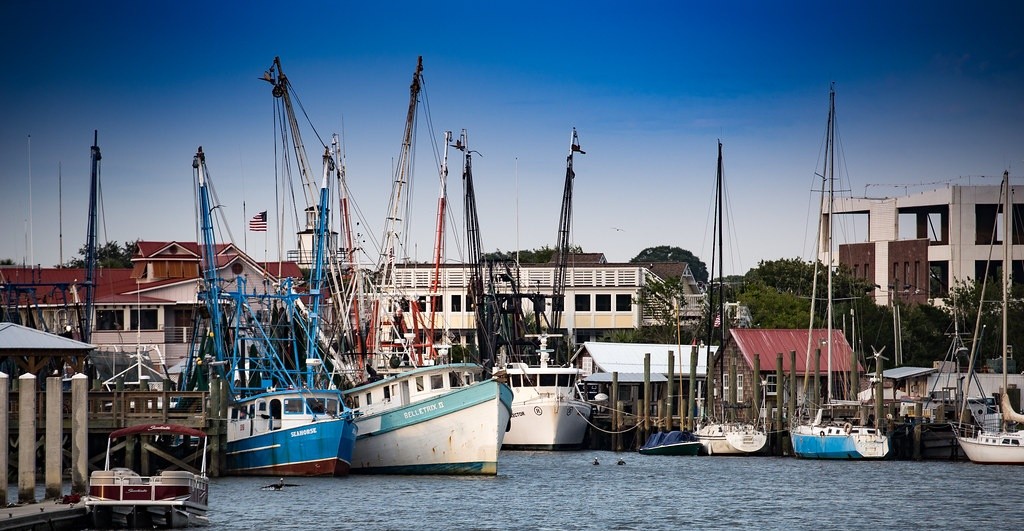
<box><xmin>249</xmin><ymin>209</ymin><xmax>268</xmax><ymax>232</ymax></box>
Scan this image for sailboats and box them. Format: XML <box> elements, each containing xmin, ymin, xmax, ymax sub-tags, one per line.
<box><xmin>950</xmin><ymin>165</ymin><xmax>1024</xmax><ymax>465</ymax></box>
<box><xmin>189</xmin><ymin>149</ymin><xmax>360</xmax><ymax>481</ymax></box>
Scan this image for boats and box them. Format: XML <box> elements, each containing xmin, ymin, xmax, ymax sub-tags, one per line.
<box><xmin>692</xmin><ymin>421</ymin><xmax>769</xmax><ymax>457</ymax></box>
<box><xmin>340</xmin><ymin>365</ymin><xmax>514</xmax><ymax>480</ymax></box>
<box><xmin>645</xmin><ymin>431</ymin><xmax>705</xmax><ymax>457</ymax></box>
<box><xmin>81</xmin><ymin>421</ymin><xmax>214</xmax><ymax>530</ymax></box>
<box><xmin>789</xmin><ymin>420</ymin><xmax>892</xmax><ymax>463</ymax></box>
<box><xmin>494</xmin><ymin>365</ymin><xmax>597</xmax><ymax>452</ymax></box>
